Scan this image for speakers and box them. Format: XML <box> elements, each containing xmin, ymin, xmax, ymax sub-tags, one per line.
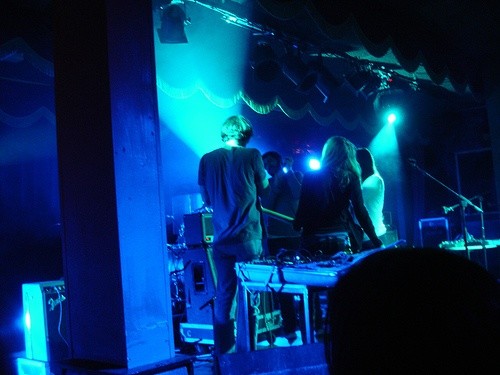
<box><xmin>183</xmin><ymin>248</ymin><xmax>218</xmax><ymax>325</ymax></box>
<box><xmin>22</xmin><ymin>280</ymin><xmax>69</xmax><ymax>363</ymax></box>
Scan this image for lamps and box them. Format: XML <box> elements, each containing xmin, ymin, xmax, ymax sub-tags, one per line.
<box><xmin>240</xmin><ymin>25</ymin><xmax>376</xmax><ymax>110</ymax></box>
<box><xmin>156</xmin><ymin>0</ymin><xmax>191</xmax><ymax>43</ymax></box>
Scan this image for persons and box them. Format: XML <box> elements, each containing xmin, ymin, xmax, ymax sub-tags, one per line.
<box><xmin>297</xmin><ymin>135</ymin><xmax>385</xmax><ymax>337</ymax></box>
<box><xmin>198</xmin><ymin>116</ymin><xmax>269</xmax><ymax>359</ymax></box>
<box><xmin>259</xmin><ymin>151</ymin><xmax>305</xmax><ymax>339</ymax></box>
<box><xmin>351</xmin><ymin>145</ymin><xmax>384</xmax><ymax>250</ymax></box>
<box><xmin>324</xmin><ymin>249</ymin><xmax>500</xmax><ymax>375</ymax></box>
